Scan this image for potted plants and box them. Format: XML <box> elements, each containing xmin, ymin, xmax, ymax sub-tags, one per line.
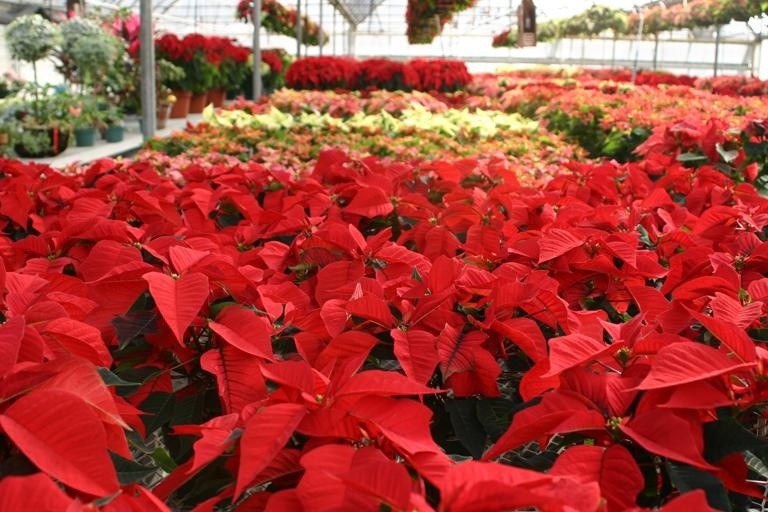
<box><xmin>0</xmin><ymin>11</ymin><xmax>132</xmax><ymax>160</ymax></box>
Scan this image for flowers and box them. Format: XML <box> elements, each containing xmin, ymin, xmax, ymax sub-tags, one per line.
<box><xmin>156</xmin><ymin>32</ymin><xmax>290</xmax><ymax>95</ymax></box>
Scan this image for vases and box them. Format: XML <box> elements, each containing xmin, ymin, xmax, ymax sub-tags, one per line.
<box><xmin>157</xmin><ymin>90</ymin><xmax>229</xmax><ymax>130</ymax></box>
